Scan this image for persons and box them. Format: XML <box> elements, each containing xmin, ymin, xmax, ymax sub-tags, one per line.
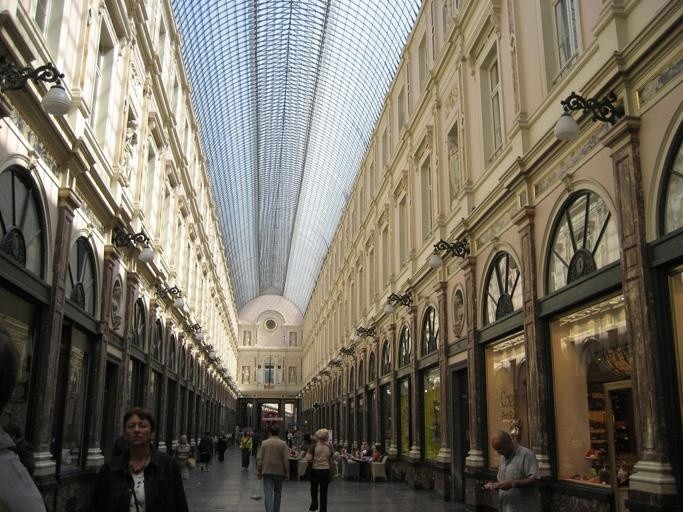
<box><xmin>0</xmin><ymin>328</ymin><xmax>47</xmax><ymax>512</ymax></box>
<box><xmin>175</xmin><ymin>427</ymin><xmax>268</xmax><ymax>480</ymax></box>
<box><xmin>304</xmin><ymin>428</ymin><xmax>336</xmax><ymax>512</ymax></box>
<box><xmin>256</xmin><ymin>423</ymin><xmax>291</xmax><ymax>512</ymax></box>
<box><xmin>332</xmin><ymin>441</ymin><xmax>382</xmax><ymax>483</ymax></box>
<box><xmin>480</xmin><ymin>430</ymin><xmax>542</xmax><ymax>512</ymax></box>
<box><xmin>95</xmin><ymin>406</ymin><xmax>189</xmax><ymax>512</ymax></box>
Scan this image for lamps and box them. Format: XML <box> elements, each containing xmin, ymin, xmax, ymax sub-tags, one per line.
<box><xmin>425</xmin><ymin>239</ymin><xmax>469</xmax><ymax>271</ymax></box>
<box><xmin>0</xmin><ymin>55</ymin><xmax>72</xmax><ymax>118</ymax></box>
<box><xmin>116</xmin><ymin>233</ymin><xmax>155</xmax><ymax>263</ymax></box>
<box><xmin>551</xmin><ymin>92</ymin><xmax>624</xmax><ymax>138</ymax></box>
<box><xmin>349</xmin><ymin>293</ymin><xmax>411</xmax><ymax>344</ymax></box>
<box><xmin>154</xmin><ymin>282</ymin><xmax>204</xmax><ymax>344</ymax></box>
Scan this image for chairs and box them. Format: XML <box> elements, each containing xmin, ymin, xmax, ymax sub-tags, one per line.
<box><xmin>332</xmin><ymin>455</ymin><xmax>389</xmax><ymax>483</ymax></box>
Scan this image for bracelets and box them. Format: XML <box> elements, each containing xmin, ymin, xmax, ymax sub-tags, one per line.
<box><xmin>511</xmin><ymin>478</ymin><xmax>515</xmax><ymax>488</ymax></box>
